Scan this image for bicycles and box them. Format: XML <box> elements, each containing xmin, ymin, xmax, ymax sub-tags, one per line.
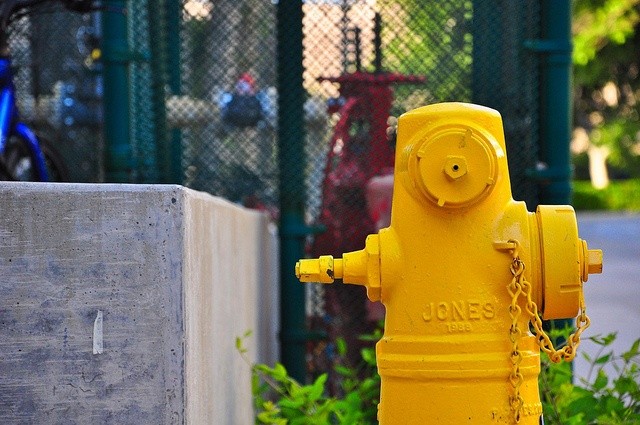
<box><xmin>1</xmin><ymin>2</ymin><xmax>94</xmax><ymax>182</ymax></box>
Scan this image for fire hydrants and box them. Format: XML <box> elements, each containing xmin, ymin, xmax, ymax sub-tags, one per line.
<box><xmin>294</xmin><ymin>100</ymin><xmax>603</xmax><ymax>424</ymax></box>
